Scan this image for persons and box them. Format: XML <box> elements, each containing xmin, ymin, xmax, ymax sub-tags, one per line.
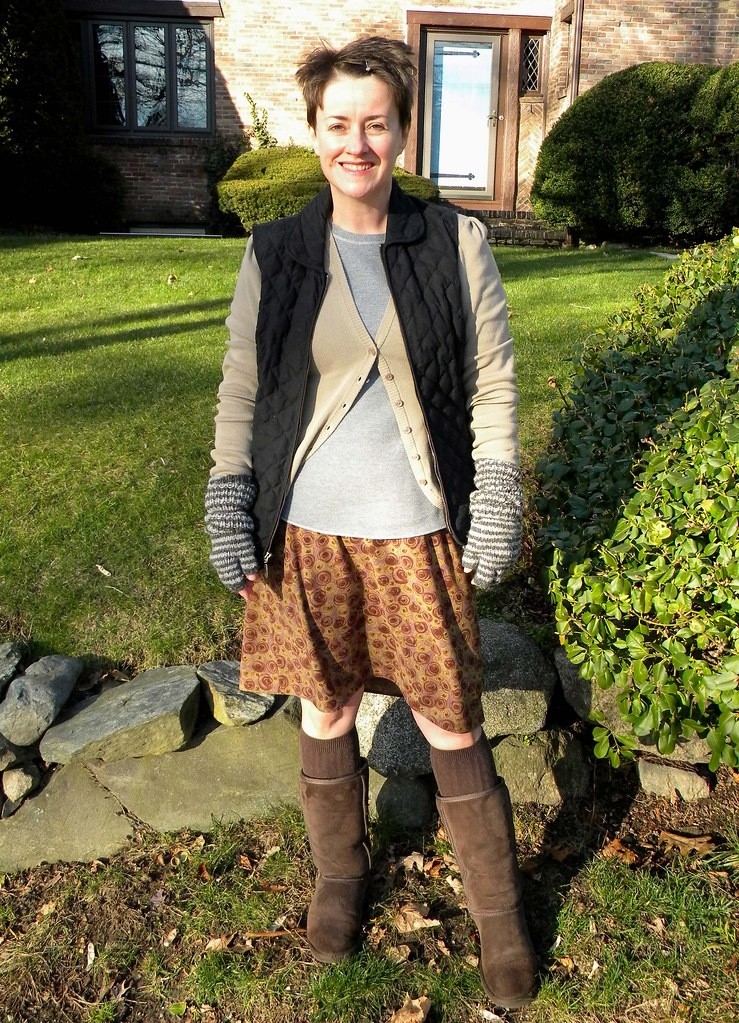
<box><xmin>201</xmin><ymin>38</ymin><xmax>542</xmax><ymax>1009</ymax></box>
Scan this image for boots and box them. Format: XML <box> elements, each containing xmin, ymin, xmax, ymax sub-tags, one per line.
<box><xmin>298</xmin><ymin>755</ymin><xmax>373</xmax><ymax>962</ymax></box>
<box><xmin>433</xmin><ymin>775</ymin><xmax>536</xmax><ymax>1007</ymax></box>
